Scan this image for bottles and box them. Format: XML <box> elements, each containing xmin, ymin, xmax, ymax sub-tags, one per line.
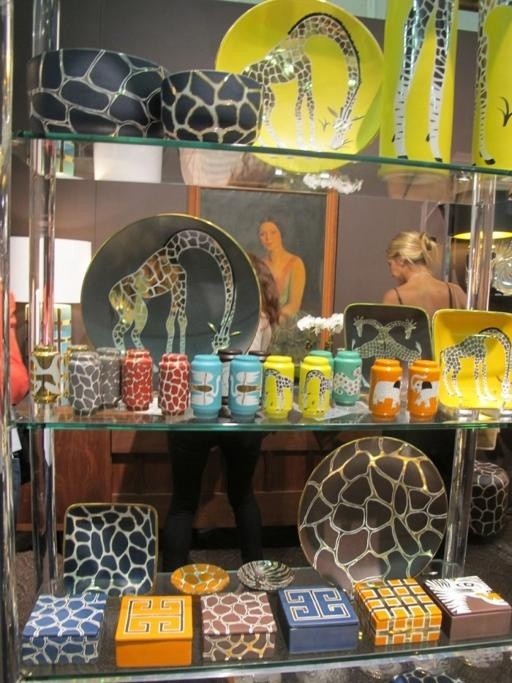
<box><xmin>188</xmin><ymin>354</ymin><xmax>224</xmax><ymax>417</ymax></box>
<box><xmin>407</xmin><ymin>360</ymin><xmax>437</xmax><ymax>416</ymax></box>
<box><xmin>29</xmin><ymin>346</ymin><xmax>87</xmax><ymax>405</ymax></box>
<box><xmin>299</xmin><ymin>355</ymin><xmax>331</xmax><ymax>421</ymax></box>
<box><xmin>30</xmin><ymin>345</ymin><xmax>439</xmax><ymax>422</ymax></box>
<box><xmin>121</xmin><ymin>349</ymin><xmax>153</xmax><ymax>411</ymax></box>
<box><xmin>69</xmin><ymin>347</ymin><xmax>121</xmax><ymax>416</ymax></box>
<box><xmin>157</xmin><ymin>353</ymin><xmax>190</xmax><ymax>416</ymax></box>
<box><xmin>229</xmin><ymin>355</ymin><xmax>261</xmax><ymax>422</ymax></box>
<box><xmin>308</xmin><ymin>350</ymin><xmax>363</xmax><ymax>405</ymax></box>
<box><xmin>262</xmin><ymin>355</ymin><xmax>294</xmax><ymax>420</ymax></box>
<box><xmin>369</xmin><ymin>359</ymin><xmax>403</xmax><ymax>417</ymax></box>
<box><xmin>217</xmin><ymin>349</ymin><xmax>273</xmax><ymax>406</ymax></box>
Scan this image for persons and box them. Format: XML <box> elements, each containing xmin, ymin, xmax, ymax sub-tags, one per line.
<box><xmin>383</xmin><ymin>232</ymin><xmax>467</xmax><ymax>461</ymax></box>
<box><xmin>162</xmin><ymin>255</ymin><xmax>279</xmax><ymax>570</ymax></box>
<box><xmin>256</xmin><ymin>217</ymin><xmax>305</xmax><ymax>326</ymax></box>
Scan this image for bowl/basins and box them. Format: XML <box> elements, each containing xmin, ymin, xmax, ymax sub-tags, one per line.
<box><xmin>160</xmin><ymin>70</ymin><xmax>274</xmax><ymax>144</ymax></box>
<box><xmin>26</xmin><ymin>48</ymin><xmax>169</xmax><ymax>138</ymax></box>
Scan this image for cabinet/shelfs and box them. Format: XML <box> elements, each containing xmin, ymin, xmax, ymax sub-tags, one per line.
<box><xmin>0</xmin><ymin>1</ymin><xmax>512</xmax><ymax>683</ymax></box>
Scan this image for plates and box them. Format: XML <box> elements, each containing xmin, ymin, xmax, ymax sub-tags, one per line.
<box><xmin>297</xmin><ymin>438</ymin><xmax>449</xmax><ymax>591</ymax></box>
<box><xmin>344</xmin><ymin>302</ymin><xmax>512</xmax><ymax>411</ymax></box>
<box><xmin>79</xmin><ymin>211</ymin><xmax>262</xmax><ymax>392</ymax></box>
<box><xmin>169</xmin><ymin>562</ymin><xmax>230</xmax><ymax>592</ymax></box>
<box><xmin>215</xmin><ymin>0</ymin><xmax>384</xmax><ymax>172</ymax></box>
<box><xmin>60</xmin><ymin>503</ymin><xmax>159</xmax><ymax>595</ymax></box>
<box><xmin>237</xmin><ymin>560</ymin><xmax>295</xmax><ymax>591</ymax></box>
